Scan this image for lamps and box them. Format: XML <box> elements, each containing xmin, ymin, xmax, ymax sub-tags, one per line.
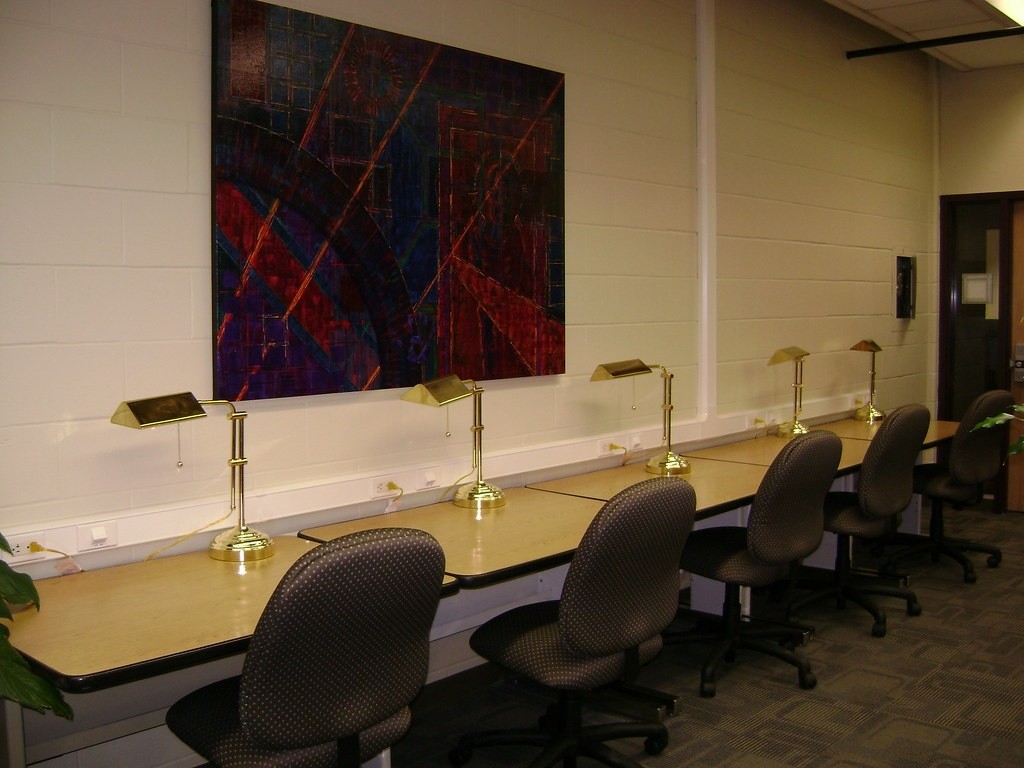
<box><xmin>767</xmin><ymin>345</ymin><xmax>811</xmax><ymax>437</ymax></box>
<box><xmin>109</xmin><ymin>392</ymin><xmax>276</xmax><ymax>566</ymax></box>
<box><xmin>588</xmin><ymin>358</ymin><xmax>691</xmax><ymax>475</ymax></box>
<box><xmin>400</xmin><ymin>374</ymin><xmax>506</xmax><ymax>509</ymax></box>
<box><xmin>849</xmin><ymin>338</ymin><xmax>886</xmax><ymax>422</ymax></box>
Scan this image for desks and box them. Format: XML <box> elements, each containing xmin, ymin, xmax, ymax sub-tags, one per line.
<box><xmin>526</xmin><ymin>455</ymin><xmax>770</xmax><ymax>522</ymax></box>
<box><xmin>811</xmin><ymin>416</ymin><xmax>960</xmax><ymax>450</ymax></box>
<box><xmin>298</xmin><ymin>486</ymin><xmax>607</xmax><ymax>588</ymax></box>
<box><xmin>680</xmin><ymin>433</ymin><xmax>873</xmax><ymax>481</ymax></box>
<box><xmin>0</xmin><ymin>534</ymin><xmax>324</xmax><ymax>768</ymax></box>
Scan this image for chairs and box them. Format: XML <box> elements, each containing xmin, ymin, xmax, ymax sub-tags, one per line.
<box><xmin>165</xmin><ymin>526</ymin><xmax>447</xmax><ymax>768</ymax></box>
<box><xmin>790</xmin><ymin>403</ymin><xmax>931</xmax><ymax>639</ymax></box>
<box><xmin>447</xmin><ymin>473</ymin><xmax>698</xmax><ymax>768</ymax></box>
<box><xmin>889</xmin><ymin>390</ymin><xmax>1017</xmax><ymax>584</ymax></box>
<box><xmin>663</xmin><ymin>429</ymin><xmax>844</xmax><ymax>698</ymax></box>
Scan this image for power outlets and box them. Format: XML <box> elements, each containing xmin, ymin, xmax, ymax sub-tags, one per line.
<box><xmin>369</xmin><ymin>474</ymin><xmax>400</xmax><ymax>501</ymax></box>
<box><xmin>0</xmin><ymin>531</ymin><xmax>45</xmax><ymax>564</ymax></box>
<box><xmin>745</xmin><ymin>414</ymin><xmax>764</xmax><ymax>431</ymax></box>
<box><xmin>850</xmin><ymin>396</ymin><xmax>865</xmax><ymax>408</ymax></box>
<box><xmin>597</xmin><ymin>437</ymin><xmax>616</xmax><ymax>456</ymax></box>
